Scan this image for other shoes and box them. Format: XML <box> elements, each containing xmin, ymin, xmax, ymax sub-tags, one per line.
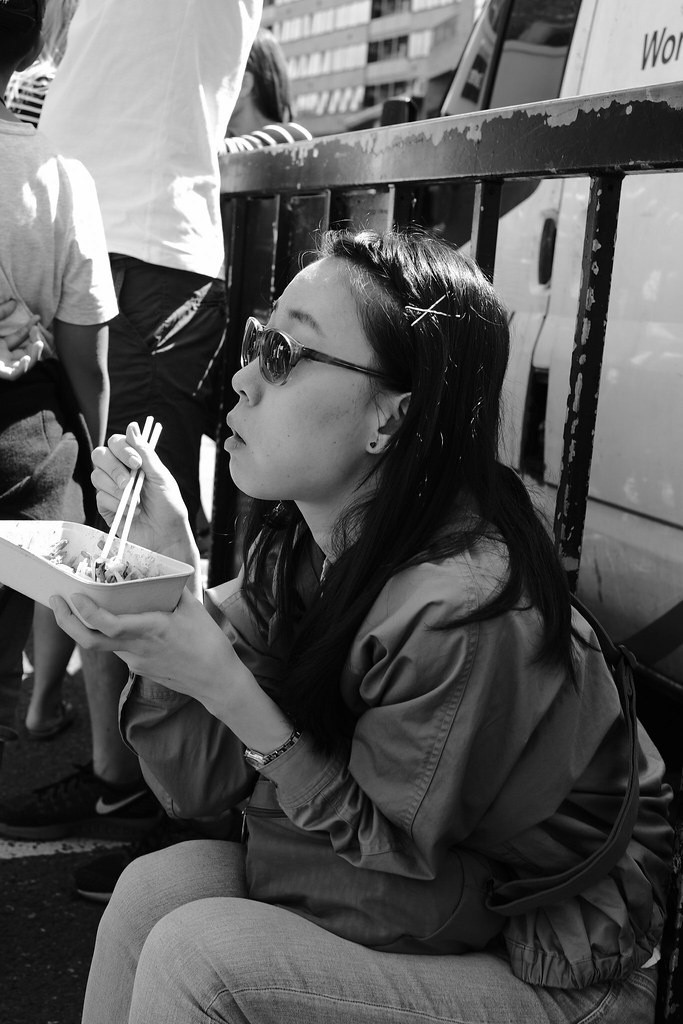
<box><xmin>74</xmin><ymin>823</ymin><xmax>169</xmax><ymax>900</ymax></box>
<box><xmin>0</xmin><ymin>759</ymin><xmax>103</xmax><ymax>841</ymax></box>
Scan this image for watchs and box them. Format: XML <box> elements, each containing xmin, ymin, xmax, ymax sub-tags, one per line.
<box><xmin>244</xmin><ymin>730</ymin><xmax>300</xmax><ymax>770</ymax></box>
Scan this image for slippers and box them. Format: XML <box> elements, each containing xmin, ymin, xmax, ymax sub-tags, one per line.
<box><xmin>30</xmin><ymin>702</ymin><xmax>74</xmax><ymax>739</ymax></box>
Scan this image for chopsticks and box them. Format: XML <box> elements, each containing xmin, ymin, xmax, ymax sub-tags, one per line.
<box><xmin>98</xmin><ymin>416</ymin><xmax>163</xmax><ymax>561</ymax></box>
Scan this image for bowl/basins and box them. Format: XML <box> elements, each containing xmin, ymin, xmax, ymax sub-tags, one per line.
<box><xmin>0</xmin><ymin>520</ymin><xmax>195</xmax><ymax>631</ymax></box>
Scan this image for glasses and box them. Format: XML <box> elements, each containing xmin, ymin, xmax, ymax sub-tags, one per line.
<box><xmin>239</xmin><ymin>315</ymin><xmax>391</xmax><ymax>385</ymax></box>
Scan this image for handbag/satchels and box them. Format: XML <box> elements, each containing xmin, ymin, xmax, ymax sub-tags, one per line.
<box><xmin>239</xmin><ymin>773</ymin><xmax>510</xmax><ymax>956</ymax></box>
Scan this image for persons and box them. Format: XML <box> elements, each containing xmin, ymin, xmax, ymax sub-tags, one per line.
<box><xmin>219</xmin><ymin>30</ymin><xmax>314</xmax><ymax>308</ymax></box>
<box><xmin>4</xmin><ymin>0</ymin><xmax>80</xmax><ymax>128</ymax></box>
<box><xmin>81</xmin><ymin>232</ymin><xmax>679</xmax><ymax>1024</ymax></box>
<box><xmin>0</xmin><ymin>0</ymin><xmax>120</xmax><ymax>737</ymax></box>
<box><xmin>0</xmin><ymin>0</ymin><xmax>263</xmax><ymax>902</ymax></box>
